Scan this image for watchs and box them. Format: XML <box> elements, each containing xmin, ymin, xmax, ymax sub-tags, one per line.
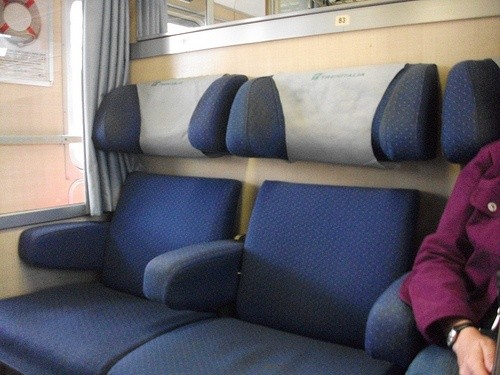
<box><xmin>446</xmin><ymin>322</ymin><xmax>481</xmax><ymax>346</ymax></box>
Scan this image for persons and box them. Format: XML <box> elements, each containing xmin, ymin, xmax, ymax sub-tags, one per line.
<box><xmin>399</xmin><ymin>141</ymin><xmax>500</xmax><ymax>375</ymax></box>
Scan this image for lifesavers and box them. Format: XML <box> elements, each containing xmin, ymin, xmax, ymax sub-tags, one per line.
<box><xmin>0</xmin><ymin>0</ymin><xmax>41</xmax><ymax>46</ymax></box>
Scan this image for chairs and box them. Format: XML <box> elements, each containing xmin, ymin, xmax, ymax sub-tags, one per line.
<box><xmin>0</xmin><ymin>59</ymin><xmax>500</xmax><ymax>375</ymax></box>
